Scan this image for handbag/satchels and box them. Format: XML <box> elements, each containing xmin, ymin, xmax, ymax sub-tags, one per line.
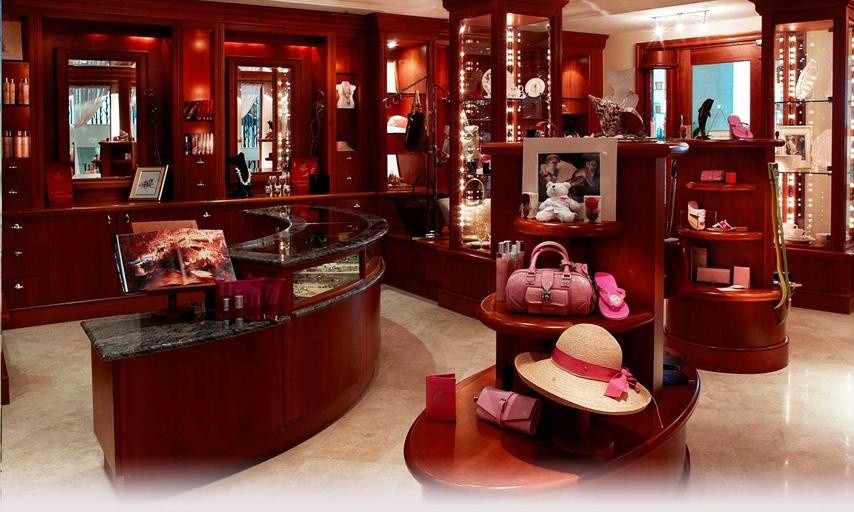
<box><xmin>403</xmin><ymin>89</ymin><xmax>430</xmax><ymax>153</ymax></box>
<box><xmin>395</xmin><ymin>172</ymin><xmax>445</xmax><ymax>238</ymax></box>
<box><xmin>505</xmin><ymin>241</ymin><xmax>594</xmax><ymax>314</ymax></box>
<box><xmin>213</xmin><ymin>274</ymin><xmax>284</xmax><ymax>323</ymax></box>
<box><xmin>441</xmin><ymin>176</ymin><xmax>493</xmax><ymax>247</ymax></box>
<box><xmin>663</xmin><ymin>232</ymin><xmax>690</xmax><ymax>301</ymax></box>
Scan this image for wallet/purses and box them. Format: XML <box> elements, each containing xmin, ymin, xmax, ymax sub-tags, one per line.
<box><xmin>693</xmin><ymin>245</ymin><xmax>749</xmax><ymax>287</ymax></box>
<box><xmin>664</xmin><ymin>353</ymin><xmax>691</xmax><ymax>385</ymax></box>
<box><xmin>701</xmin><ymin>167</ymin><xmax>723</xmax><ymax>181</ymax></box>
<box><xmin>422</xmin><ymin>372</ymin><xmax>459</xmax><ymax>419</ymax></box>
<box><xmin>475</xmin><ymin>385</ymin><xmax>539</xmax><ymax>434</ymax></box>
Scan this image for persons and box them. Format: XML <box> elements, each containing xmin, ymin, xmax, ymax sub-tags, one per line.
<box><xmin>787</xmin><ymin>136</ymin><xmax>804</xmax><ymax>154</ymax></box>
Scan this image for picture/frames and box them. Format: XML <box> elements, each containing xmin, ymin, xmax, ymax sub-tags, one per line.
<box><xmin>128</xmin><ymin>161</ymin><xmax>170</xmax><ymax>203</ymax></box>
<box><xmin>777</xmin><ymin>124</ymin><xmax>815</xmax><ymax>169</ymax></box>
<box><xmin>1</xmin><ymin>16</ymin><xmax>25</xmax><ymax>61</ymax></box>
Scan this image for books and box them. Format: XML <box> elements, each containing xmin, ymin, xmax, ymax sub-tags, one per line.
<box><xmin>116</xmin><ymin>229</ymin><xmax>236</xmax><ymax>291</ymax></box>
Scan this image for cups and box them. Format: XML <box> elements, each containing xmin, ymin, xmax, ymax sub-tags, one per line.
<box><xmin>264</xmin><ymin>172</ymin><xmax>292</xmax><ymax>195</ymax></box>
<box><xmin>781</xmin><ymin>223</ymin><xmax>814</xmax><ymax>241</ymax></box>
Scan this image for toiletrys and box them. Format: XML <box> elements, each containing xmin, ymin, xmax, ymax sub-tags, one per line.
<box><xmin>3</xmin><ymin>130</ymin><xmax>29</xmax><ymax>158</ymax></box>
<box><xmin>9</xmin><ymin>79</ymin><xmax>15</xmax><ymax>104</ymax></box>
<box><xmin>17</xmin><ymin>77</ymin><xmax>23</xmax><ymax>104</ymax></box>
<box><xmin>3</xmin><ymin>77</ymin><xmax>9</xmax><ymax>103</ymax></box>
<box><xmin>496</xmin><ymin>240</ymin><xmax>525</xmax><ymax>303</ymax></box>
<box><xmin>22</xmin><ymin>78</ymin><xmax>29</xmax><ymax>105</ymax></box>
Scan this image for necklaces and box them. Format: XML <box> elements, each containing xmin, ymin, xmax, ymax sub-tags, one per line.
<box><xmin>235</xmin><ymin>167</ymin><xmax>252</xmax><ymax>186</ymax></box>
<box><xmin>342</xmin><ymin>86</ymin><xmax>351</xmax><ymax>104</ymax></box>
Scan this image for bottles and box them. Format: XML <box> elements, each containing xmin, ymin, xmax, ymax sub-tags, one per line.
<box><xmin>677</xmin><ymin>115</ymin><xmax>687</xmax><ymax>139</ymax></box>
<box><xmin>3</xmin><ymin>76</ymin><xmax>30</xmax><ymax>105</ymax></box>
<box><xmin>495</xmin><ymin>239</ymin><xmax>525</xmax><ymax>302</ymax></box>
<box><xmin>3</xmin><ymin>129</ymin><xmax>30</xmax><ymax>159</ymax></box>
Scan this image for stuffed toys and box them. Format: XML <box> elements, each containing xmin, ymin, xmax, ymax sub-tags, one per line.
<box><xmin>535</xmin><ymin>181</ymin><xmax>581</xmax><ymax>222</ymax></box>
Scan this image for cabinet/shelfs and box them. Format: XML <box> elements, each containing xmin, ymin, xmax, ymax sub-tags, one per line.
<box><xmin>334</xmin><ymin>71</ymin><xmax>361</xmax><ymax>191</ymax></box>
<box><xmin>442</xmin><ymin>0</ymin><xmax>569</xmax><ymax>314</ymax></box>
<box><xmin>561</xmin><ymin>33</ymin><xmax>606</xmax><ymax>135</ymax></box>
<box><xmin>180</xmin><ymin>99</ymin><xmax>218</xmax><ymax>201</ymax></box>
<box><xmin>746</xmin><ymin>0</ymin><xmax>853</xmax><ymax>316</ymax></box>
<box><xmin>401</xmin><ymin>142</ymin><xmax>701</xmax><ymax>512</ymax></box>
<box><xmin>98</xmin><ymin>141</ymin><xmax>136</xmax><ymax>178</ymax></box>
<box><xmin>661</xmin><ymin>137</ymin><xmax>792</xmax><ymax>375</ymax></box>
<box><xmin>386</xmin><ymin>34</ymin><xmax>432</xmax><ymax>188</ymax></box>
<box><xmin>2</xmin><ymin>104</ymin><xmax>31</xmax><ymax>211</ymax></box>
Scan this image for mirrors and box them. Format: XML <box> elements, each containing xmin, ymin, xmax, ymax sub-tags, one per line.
<box><xmin>55</xmin><ymin>45</ymin><xmax>151</xmax><ymax>190</ymax></box>
<box><xmin>226</xmin><ymin>54</ymin><xmax>302</xmax><ymax>186</ymax></box>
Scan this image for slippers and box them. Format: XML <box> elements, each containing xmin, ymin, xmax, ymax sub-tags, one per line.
<box><xmin>687</xmin><ymin>199</ymin><xmax>706</xmax><ymax>230</ymax></box>
<box><xmin>594</xmin><ymin>269</ymin><xmax>631</xmax><ymax>322</ymax></box>
<box><xmin>706</xmin><ymin>219</ymin><xmax>745</xmax><ymax>233</ymax></box>
<box><xmin>729</xmin><ymin>114</ymin><xmax>752</xmax><ymax>139</ymax></box>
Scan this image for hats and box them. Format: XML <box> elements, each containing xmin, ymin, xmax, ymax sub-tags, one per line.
<box><xmin>512</xmin><ymin>322</ymin><xmax>658</xmax><ymax>416</ymax></box>
<box><xmin>774</xmin><ymin>270</ymin><xmax>803</xmax><ymax>287</ymax></box>
